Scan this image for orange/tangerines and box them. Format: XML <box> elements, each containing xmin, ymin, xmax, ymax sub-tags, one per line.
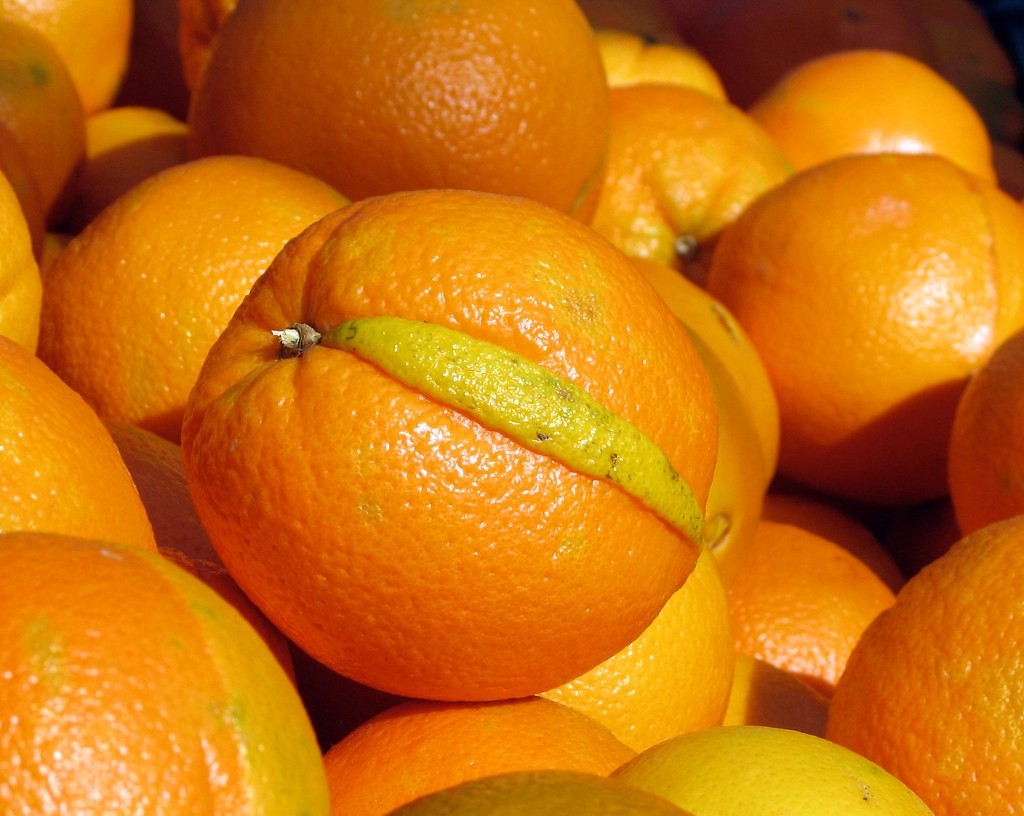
<box><xmin>0</xmin><ymin>0</ymin><xmax>1024</xmax><ymax>816</ymax></box>
